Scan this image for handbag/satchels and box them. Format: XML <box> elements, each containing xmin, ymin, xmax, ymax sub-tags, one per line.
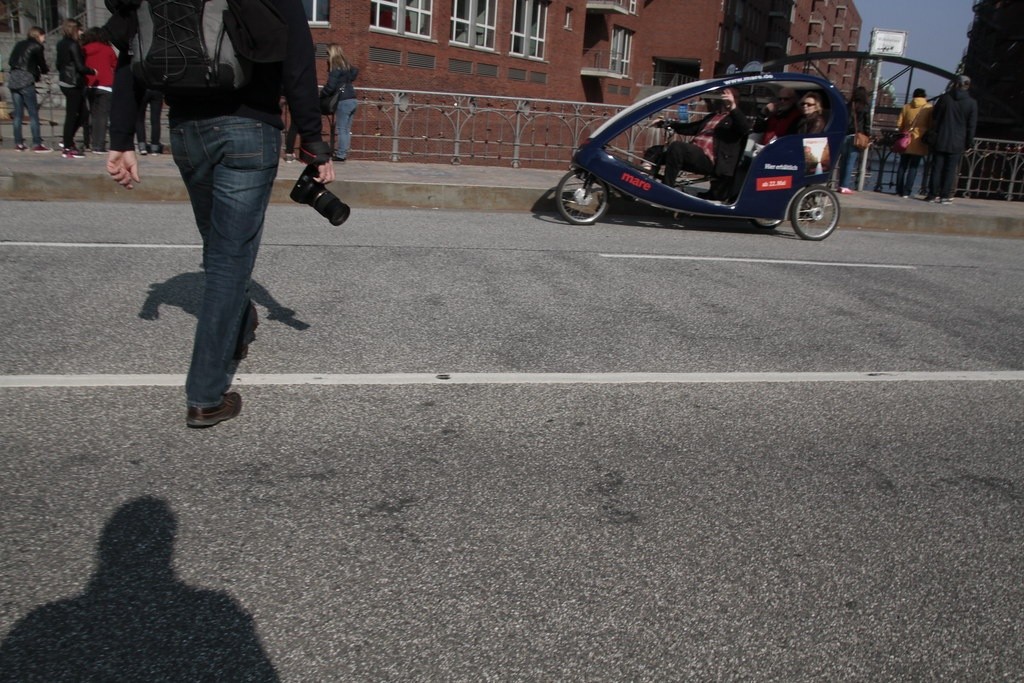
<box><xmin>854</xmin><ymin>131</ymin><xmax>869</xmax><ymax>150</ymax></box>
<box><xmin>319</xmin><ymin>91</ymin><xmax>342</xmax><ymax>116</ymax></box>
<box><xmin>893</xmin><ymin>130</ymin><xmax>912</xmax><ymax>152</ymax></box>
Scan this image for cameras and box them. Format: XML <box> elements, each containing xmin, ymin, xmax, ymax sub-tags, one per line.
<box><xmin>289</xmin><ymin>164</ymin><xmax>350</xmax><ymax>226</ymax></box>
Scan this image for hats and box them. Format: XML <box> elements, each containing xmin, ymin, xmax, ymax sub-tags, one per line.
<box><xmin>955</xmin><ymin>75</ymin><xmax>971</xmax><ymax>91</ymax></box>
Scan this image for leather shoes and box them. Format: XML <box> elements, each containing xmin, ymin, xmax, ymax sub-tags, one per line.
<box><xmin>186</xmin><ymin>390</ymin><xmax>241</xmax><ymax>426</ymax></box>
<box><xmin>233</xmin><ymin>345</ymin><xmax>249</xmax><ymax>360</ymax></box>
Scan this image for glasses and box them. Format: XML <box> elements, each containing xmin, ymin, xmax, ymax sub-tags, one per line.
<box><xmin>779</xmin><ymin>96</ymin><xmax>794</xmax><ymax>101</ymax></box>
<box><xmin>800</xmin><ymin>102</ymin><xmax>816</xmax><ymax>107</ymax></box>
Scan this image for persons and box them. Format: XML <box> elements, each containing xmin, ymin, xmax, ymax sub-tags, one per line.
<box><xmin>103</xmin><ymin>1</ymin><xmax>335</xmax><ymax>428</ymax></box>
<box><xmin>897</xmin><ymin>87</ymin><xmax>934</xmax><ymax>198</ymax></box>
<box><xmin>924</xmin><ymin>74</ymin><xmax>978</xmax><ymax>204</ymax></box>
<box><xmin>7</xmin><ymin>26</ymin><xmax>53</xmax><ymax>153</ymax></box>
<box><xmin>837</xmin><ymin>87</ymin><xmax>871</xmax><ymax>194</ymax></box>
<box><xmin>55</xmin><ymin>19</ymin><xmax>165</xmax><ymax>159</ymax></box>
<box><xmin>284</xmin><ymin>43</ymin><xmax>360</xmax><ymax>161</ymax></box>
<box><xmin>729</xmin><ymin>91</ymin><xmax>826</xmax><ymax>205</ymax></box>
<box><xmin>641</xmin><ymin>86</ymin><xmax>750</xmax><ymax>189</ymax></box>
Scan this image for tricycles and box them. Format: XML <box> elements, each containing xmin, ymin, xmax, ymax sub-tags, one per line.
<box><xmin>555</xmin><ymin>71</ymin><xmax>855</xmax><ymax>241</ymax></box>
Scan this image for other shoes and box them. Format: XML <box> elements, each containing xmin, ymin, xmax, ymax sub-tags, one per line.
<box><xmin>93</xmin><ymin>149</ymin><xmax>108</xmax><ymax>156</ymax></box>
<box><xmin>149</xmin><ymin>150</ymin><xmax>160</xmax><ymax>156</ymax></box>
<box><xmin>837</xmin><ymin>186</ymin><xmax>854</xmax><ymax>194</ymax></box>
<box><xmin>940</xmin><ymin>197</ymin><xmax>952</xmax><ymax>203</ymax></box>
<box><xmin>901</xmin><ymin>194</ymin><xmax>907</xmax><ymax>198</ymax></box>
<box><xmin>332</xmin><ymin>156</ymin><xmax>345</xmax><ymax>162</ymax></box>
<box><xmin>286</xmin><ymin>154</ymin><xmax>297</xmax><ymax>162</ymax></box>
<box><xmin>141</xmin><ymin>149</ymin><xmax>147</xmax><ymax>153</ymax></box>
<box><xmin>923</xmin><ymin>195</ymin><xmax>939</xmax><ymax>202</ymax></box>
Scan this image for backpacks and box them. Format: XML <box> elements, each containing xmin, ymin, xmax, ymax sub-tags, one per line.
<box><xmin>128</xmin><ymin>0</ymin><xmax>248</xmax><ymax>92</ymax></box>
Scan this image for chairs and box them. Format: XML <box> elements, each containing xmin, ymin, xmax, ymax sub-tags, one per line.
<box><xmin>694</xmin><ymin>131</ymin><xmax>748</xmax><ymax>202</ymax></box>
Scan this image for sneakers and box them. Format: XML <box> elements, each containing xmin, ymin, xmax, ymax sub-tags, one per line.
<box><xmin>33</xmin><ymin>144</ymin><xmax>52</xmax><ymax>153</ymax></box>
<box><xmin>59</xmin><ymin>140</ymin><xmax>78</xmax><ymax>152</ymax></box>
<box><xmin>62</xmin><ymin>148</ymin><xmax>86</xmax><ymax>159</ymax></box>
<box><xmin>14</xmin><ymin>144</ymin><xmax>28</xmax><ymax>151</ymax></box>
